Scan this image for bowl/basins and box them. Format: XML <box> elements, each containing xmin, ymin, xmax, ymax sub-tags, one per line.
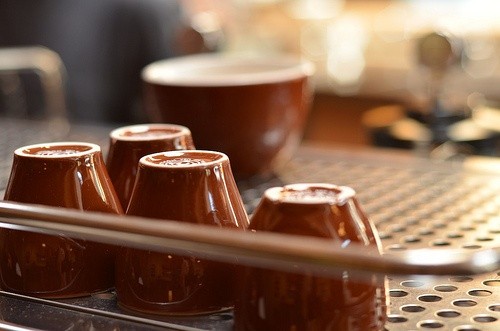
<box><xmin>140</xmin><ymin>51</ymin><xmax>316</xmax><ymax>190</ymax></box>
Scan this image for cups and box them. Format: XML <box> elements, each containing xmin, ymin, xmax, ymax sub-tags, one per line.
<box><xmin>105</xmin><ymin>122</ymin><xmax>198</xmax><ymax>214</ymax></box>
<box><xmin>231</xmin><ymin>182</ymin><xmax>391</xmax><ymax>331</ymax></box>
<box><xmin>114</xmin><ymin>148</ymin><xmax>251</xmax><ymax>316</ymax></box>
<box><xmin>0</xmin><ymin>142</ymin><xmax>126</xmax><ymax>300</ymax></box>
